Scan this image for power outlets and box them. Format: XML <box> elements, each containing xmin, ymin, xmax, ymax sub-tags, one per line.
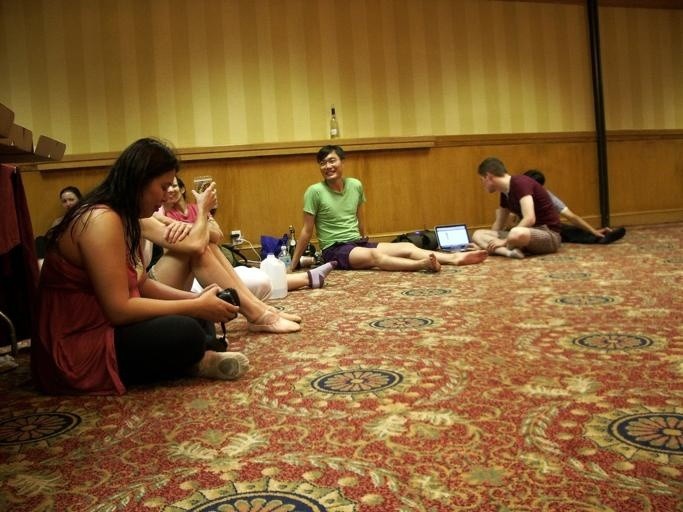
<box><xmin>229</xmin><ymin>229</ymin><xmax>243</xmax><ymax>247</ymax></box>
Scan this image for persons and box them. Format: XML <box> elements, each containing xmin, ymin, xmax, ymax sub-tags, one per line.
<box><xmin>496</xmin><ymin>170</ymin><xmax>626</xmax><ymax>244</ymax></box>
<box><xmin>288</xmin><ymin>145</ymin><xmax>488</xmax><ymax>272</ymax></box>
<box><xmin>463</xmin><ymin>158</ymin><xmax>561</xmax><ymax>259</ymax></box>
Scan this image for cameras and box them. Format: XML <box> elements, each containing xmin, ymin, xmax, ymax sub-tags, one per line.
<box><xmin>217</xmin><ymin>288</ymin><xmax>240</xmax><ymax>322</ymax></box>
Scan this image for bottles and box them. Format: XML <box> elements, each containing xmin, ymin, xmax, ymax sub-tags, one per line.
<box><xmin>286</xmin><ymin>223</ymin><xmax>300</xmax><ymax>270</ymax></box>
<box><xmin>329</xmin><ymin>107</ymin><xmax>339</xmax><ymax>140</ymax></box>
<box><xmin>278</xmin><ymin>245</ymin><xmax>291</xmax><ymax>273</ymax></box>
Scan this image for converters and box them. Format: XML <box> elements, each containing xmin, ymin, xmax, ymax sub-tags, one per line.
<box><xmin>231</xmin><ymin>234</ymin><xmax>239</xmax><ymax>237</ymax></box>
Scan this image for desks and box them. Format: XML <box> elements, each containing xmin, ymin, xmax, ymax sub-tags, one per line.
<box><xmin>0</xmin><ymin>160</ymin><xmax>47</xmax><ymax>384</ymax></box>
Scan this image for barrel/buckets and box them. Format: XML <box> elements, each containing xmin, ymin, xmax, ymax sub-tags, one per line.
<box><xmin>259</xmin><ymin>252</ymin><xmax>289</xmax><ymax>300</ymax></box>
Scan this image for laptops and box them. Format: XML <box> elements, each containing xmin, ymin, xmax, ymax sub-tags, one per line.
<box><xmin>434</xmin><ymin>223</ymin><xmax>475</xmax><ymax>253</ymax></box>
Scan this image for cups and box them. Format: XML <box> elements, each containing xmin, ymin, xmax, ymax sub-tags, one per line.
<box><xmin>299</xmin><ymin>256</ymin><xmax>316</xmax><ymax>267</ymax></box>
